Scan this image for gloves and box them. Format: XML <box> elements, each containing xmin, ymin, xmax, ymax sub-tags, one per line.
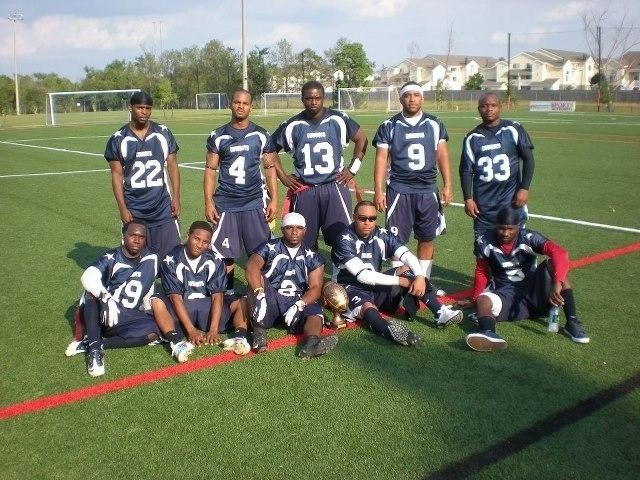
<box><xmin>281</xmin><ymin>300</ymin><xmax>305</xmax><ymax>330</ymax></box>
<box><xmin>250</xmin><ymin>287</ymin><xmax>267</xmax><ymax>323</ymax></box>
<box><xmin>99</xmin><ymin>291</ymin><xmax>124</xmax><ymax>327</ymax></box>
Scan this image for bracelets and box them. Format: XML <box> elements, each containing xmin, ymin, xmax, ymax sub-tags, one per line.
<box><xmin>253</xmin><ymin>287</ymin><xmax>265</xmax><ymax>293</ymax></box>
<box><xmin>347</xmin><ymin>157</ymin><xmax>362</xmax><ymax>177</ymax></box>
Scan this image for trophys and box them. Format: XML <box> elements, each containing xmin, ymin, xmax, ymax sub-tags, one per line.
<box><xmin>322</xmin><ymin>281</ymin><xmax>350</xmax><ymax>331</ymax></box>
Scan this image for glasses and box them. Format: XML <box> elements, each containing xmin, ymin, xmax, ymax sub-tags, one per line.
<box><xmin>355</xmin><ymin>215</ymin><xmax>376</xmax><ymax>222</ymax></box>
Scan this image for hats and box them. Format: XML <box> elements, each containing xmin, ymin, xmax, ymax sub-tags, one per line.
<box><xmin>497</xmin><ymin>208</ymin><xmax>519</xmax><ymax>225</ymax></box>
<box><xmin>131</xmin><ymin>91</ymin><xmax>153</xmax><ymax>106</ymax></box>
<box><xmin>283</xmin><ymin>212</ymin><xmax>306</xmax><ymax>227</ymax></box>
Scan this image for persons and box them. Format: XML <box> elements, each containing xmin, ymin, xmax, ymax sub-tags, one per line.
<box><xmin>372</xmin><ymin>81</ymin><xmax>454</xmax><ymax>280</ymax></box>
<box><xmin>103</xmin><ymin>91</ymin><xmax>182</xmax><ymax>277</ymax></box>
<box><xmin>458</xmin><ymin>92</ymin><xmax>535</xmax><ymax>260</ymax></box>
<box><xmin>148</xmin><ymin>220</ymin><xmax>252</xmax><ymax>363</ymax></box>
<box><xmin>330</xmin><ymin>200</ymin><xmax>463</xmax><ymax>347</ymax></box>
<box><xmin>271</xmin><ymin>80</ymin><xmax>369</xmax><ymax>282</ymax></box>
<box><xmin>203</xmin><ymin>87</ymin><xmax>278</xmax><ymax>295</ymax></box>
<box><xmin>244</xmin><ymin>211</ymin><xmax>339</xmax><ymax>359</ymax></box>
<box><xmin>456</xmin><ymin>208</ymin><xmax>590</xmax><ymax>351</ymax></box>
<box><xmin>64</xmin><ymin>222</ymin><xmax>163</xmax><ymax>376</ymax></box>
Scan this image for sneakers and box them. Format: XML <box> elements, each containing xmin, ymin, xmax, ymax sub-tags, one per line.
<box><xmin>425</xmin><ymin>277</ymin><xmax>432</xmax><ymax>292</ymax></box>
<box><xmin>466</xmin><ymin>332</ymin><xmax>508</xmax><ymax>351</ymax></box>
<box><xmin>563</xmin><ymin>319</ymin><xmax>590</xmax><ymax>343</ymax></box>
<box><xmin>64</xmin><ymin>335</ymin><xmax>89</xmax><ymax>356</ymax></box>
<box><xmin>223</xmin><ymin>337</ymin><xmax>251</xmax><ymax>355</ymax></box>
<box><xmin>88</xmin><ymin>348</ymin><xmax>105</xmax><ymax>377</ymax></box>
<box><xmin>298</xmin><ymin>336</ymin><xmax>338</xmax><ymax>357</ymax></box>
<box><xmin>253</xmin><ymin>327</ymin><xmax>267</xmax><ymax>354</ymax></box>
<box><xmin>387</xmin><ymin>321</ymin><xmax>421</xmax><ymax>346</ymax></box>
<box><xmin>172</xmin><ymin>341</ymin><xmax>194</xmax><ymax>362</ymax></box>
<box><xmin>433</xmin><ymin>304</ymin><xmax>464</xmax><ymax>328</ymax></box>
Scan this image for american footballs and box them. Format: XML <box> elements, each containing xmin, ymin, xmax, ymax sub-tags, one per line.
<box><xmin>322</xmin><ymin>281</ymin><xmax>349</xmax><ymax>312</ymax></box>
